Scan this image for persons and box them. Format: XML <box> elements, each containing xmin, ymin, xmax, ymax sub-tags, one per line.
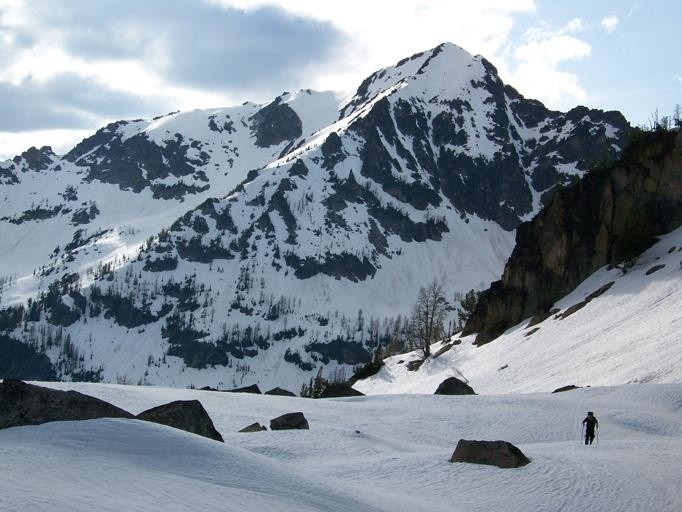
<box><xmin>583</xmin><ymin>412</ymin><xmax>598</xmax><ymax>445</ymax></box>
